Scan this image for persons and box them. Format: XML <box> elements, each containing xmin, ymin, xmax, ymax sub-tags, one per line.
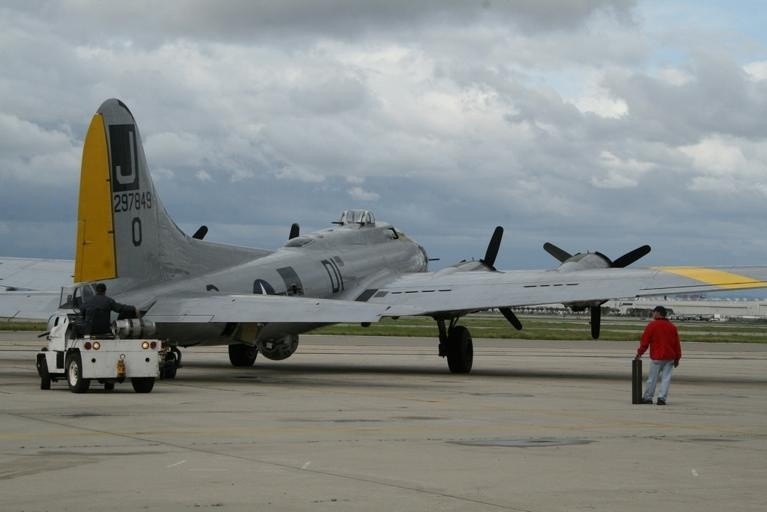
<box><xmin>79</xmin><ymin>283</ymin><xmax>136</xmax><ymax>338</ymax></box>
<box><xmin>634</xmin><ymin>305</ymin><xmax>682</xmax><ymax>406</ymax></box>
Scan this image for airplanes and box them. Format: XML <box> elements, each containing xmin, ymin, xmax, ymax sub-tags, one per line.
<box><xmin>0</xmin><ymin>97</ymin><xmax>767</xmax><ymax>397</ymax></box>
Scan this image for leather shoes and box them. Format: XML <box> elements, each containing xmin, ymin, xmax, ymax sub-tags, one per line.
<box><xmin>642</xmin><ymin>398</ymin><xmax>652</xmax><ymax>404</ymax></box>
<box><xmin>658</xmin><ymin>400</ymin><xmax>665</xmax><ymax>405</ymax></box>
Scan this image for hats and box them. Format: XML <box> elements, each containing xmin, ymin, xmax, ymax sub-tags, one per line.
<box><xmin>650</xmin><ymin>306</ymin><xmax>667</xmax><ymax>316</ymax></box>
<box><xmin>96</xmin><ymin>284</ymin><xmax>106</xmax><ymax>292</ymax></box>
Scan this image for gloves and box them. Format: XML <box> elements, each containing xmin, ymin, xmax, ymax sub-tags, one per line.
<box><xmin>674</xmin><ymin>360</ymin><xmax>678</xmax><ymax>368</ymax></box>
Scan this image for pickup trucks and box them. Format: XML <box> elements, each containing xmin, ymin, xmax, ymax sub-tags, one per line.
<box><xmin>35</xmin><ymin>282</ymin><xmax>172</xmax><ymax>393</ymax></box>
